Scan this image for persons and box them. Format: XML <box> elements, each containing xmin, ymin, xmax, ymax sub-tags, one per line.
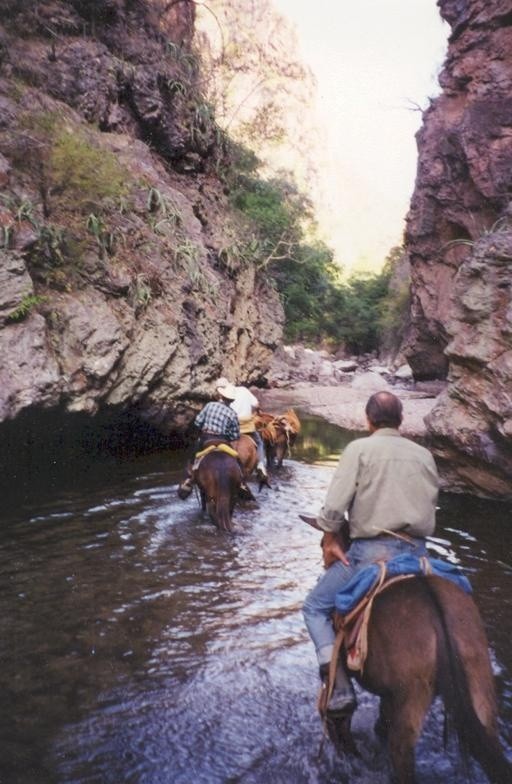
<box><xmin>302</xmin><ymin>391</ymin><xmax>440</xmax><ymax>718</ymax></box>
<box><xmin>215</xmin><ymin>378</ymin><xmax>268</xmax><ymax>481</ymax></box>
<box><xmin>184</xmin><ymin>384</ymin><xmax>248</xmax><ymax>493</ymax></box>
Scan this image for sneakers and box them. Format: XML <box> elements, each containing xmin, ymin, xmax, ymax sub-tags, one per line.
<box><xmin>321</xmin><ymin>685</ymin><xmax>359</xmax><ymax>714</ymax></box>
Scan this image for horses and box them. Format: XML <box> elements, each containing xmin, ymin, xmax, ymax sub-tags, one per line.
<box><xmin>194</xmin><ymin>406</ymin><xmax>301</xmax><ymax>533</ymax></box>
<box><xmin>298</xmin><ymin>514</ymin><xmax>511</xmax><ymax>784</ymax></box>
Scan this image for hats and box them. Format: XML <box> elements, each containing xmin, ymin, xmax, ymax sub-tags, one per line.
<box><xmin>216</xmin><ymin>384</ymin><xmax>238</xmax><ymax>401</ymax></box>
